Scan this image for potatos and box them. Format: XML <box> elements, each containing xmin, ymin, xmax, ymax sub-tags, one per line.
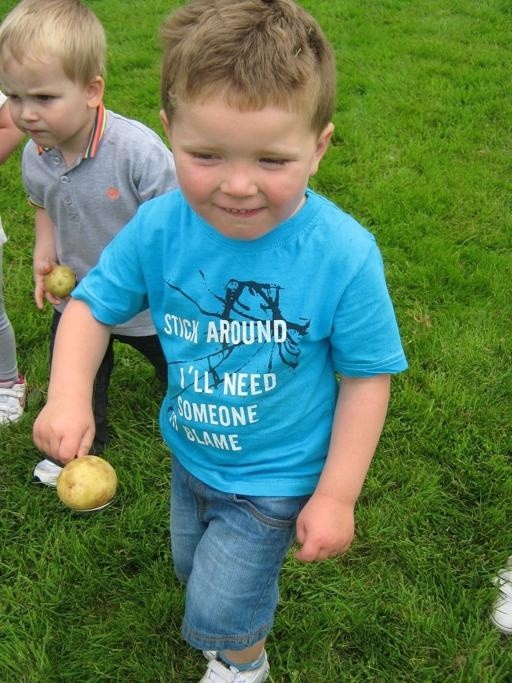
<box><xmin>55</xmin><ymin>455</ymin><xmax>118</xmax><ymax>512</ymax></box>
<box><xmin>43</xmin><ymin>266</ymin><xmax>77</xmax><ymax>299</ymax></box>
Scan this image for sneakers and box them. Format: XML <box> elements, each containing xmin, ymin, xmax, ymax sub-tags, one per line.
<box><xmin>200</xmin><ymin>649</ymin><xmax>271</xmax><ymax>683</ymax></box>
<box><xmin>33</xmin><ymin>459</ymin><xmax>62</xmax><ymax>488</ymax></box>
<box><xmin>489</xmin><ymin>556</ymin><xmax>512</xmax><ymax>632</ymax></box>
<box><xmin>0</xmin><ymin>373</ymin><xmax>27</xmax><ymax>425</ymax></box>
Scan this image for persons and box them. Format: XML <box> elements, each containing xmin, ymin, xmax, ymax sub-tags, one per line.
<box><xmin>0</xmin><ymin>0</ymin><xmax>180</xmax><ymax>488</ymax></box>
<box><xmin>32</xmin><ymin>1</ymin><xmax>408</xmax><ymax>682</ymax></box>
<box><xmin>0</xmin><ymin>84</ymin><xmax>29</xmax><ymax>427</ymax></box>
